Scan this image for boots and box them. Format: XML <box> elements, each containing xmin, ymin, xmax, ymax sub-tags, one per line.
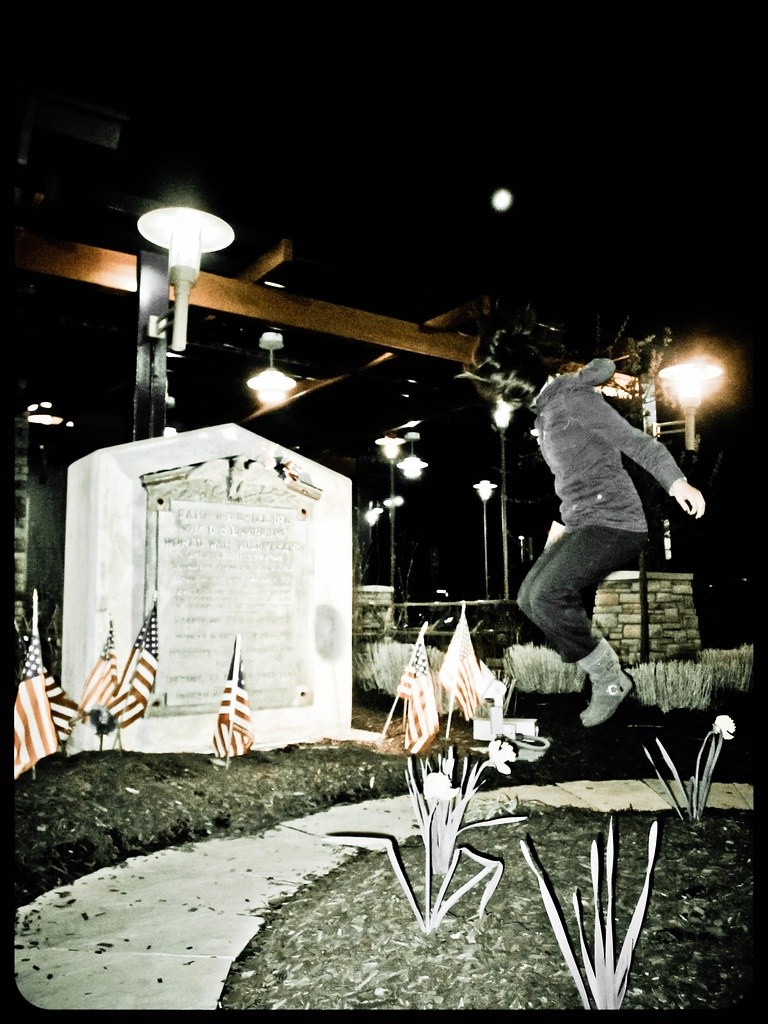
<box><xmin>576</xmin><ymin>637</ymin><xmax>632</xmax><ymax>727</ymax></box>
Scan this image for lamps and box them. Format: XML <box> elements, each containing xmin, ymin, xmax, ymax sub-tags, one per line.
<box><xmin>247</xmin><ymin>349</ymin><xmax>297</xmax><ymax>406</ymax></box>
<box><xmin>650</xmin><ymin>354</ymin><xmax>724</xmax><ymax>456</ymax></box>
<box><xmin>396</xmin><ymin>440</ymin><xmax>428</xmax><ymax>479</ymax></box>
<box><xmin>136</xmin><ymin>177</ymin><xmax>236</xmax><ymax>352</ymax></box>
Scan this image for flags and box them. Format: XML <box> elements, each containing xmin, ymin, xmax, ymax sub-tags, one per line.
<box><xmin>75</xmin><ymin>620</ymin><xmax>119</xmax><ymax>722</ymax></box>
<box><xmin>213</xmin><ymin>636</ymin><xmax>257</xmax><ymax>759</ymax></box>
<box><xmin>435</xmin><ymin>607</ymin><xmax>488</xmax><ymax>721</ymax></box>
<box><xmin>13</xmin><ymin>610</ymin><xmax>63</xmax><ymax>779</ymax></box>
<box><xmin>103</xmin><ymin>601</ymin><xmax>161</xmax><ymax>731</ymax></box>
<box><xmin>14</xmin><ymin>617</ymin><xmax>87</xmax><ymax>749</ymax></box>
<box><xmin>394</xmin><ymin>632</ymin><xmax>442</xmax><ymax>755</ymax></box>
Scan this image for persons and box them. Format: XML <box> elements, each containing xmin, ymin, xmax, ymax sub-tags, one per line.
<box><xmin>468</xmin><ymin>324</ymin><xmax>707</xmax><ymax>728</ymax></box>
